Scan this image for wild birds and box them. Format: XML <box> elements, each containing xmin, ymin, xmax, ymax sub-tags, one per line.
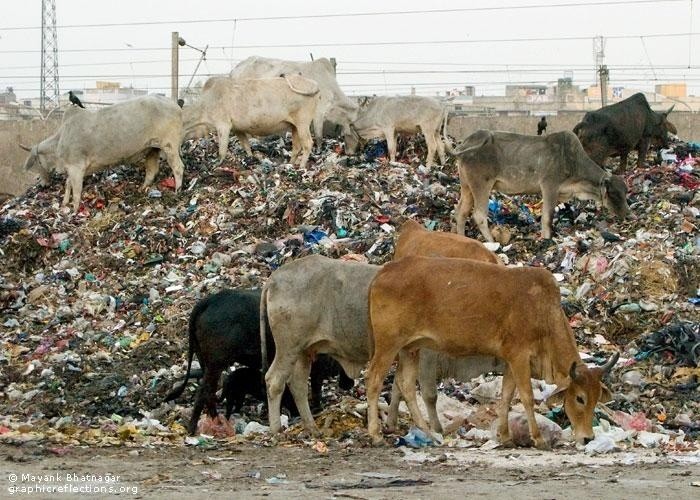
<box><xmin>68</xmin><ymin>90</ymin><xmax>86</xmax><ymax>110</ymax></box>
<box><xmin>537</xmin><ymin>116</ymin><xmax>548</xmax><ymax>135</ymax></box>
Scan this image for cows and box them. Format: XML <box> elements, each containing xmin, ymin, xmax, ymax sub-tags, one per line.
<box><xmin>18</xmin><ymin>94</ymin><xmax>186</xmax><ymax>207</ymax></box>
<box><xmin>230</xmin><ymin>54</ymin><xmax>359</xmax><ymax>141</ymax></box>
<box><xmin>178</xmin><ymin>74</ymin><xmax>320</xmax><ymax>168</ymax></box>
<box><xmin>573</xmin><ymin>92</ymin><xmax>678</xmax><ymax>174</ymax></box>
<box><xmin>453</xmin><ymin>129</ymin><xmax>634</xmax><ymax>243</ymax></box>
<box><xmin>340</xmin><ymin>94</ymin><xmax>450</xmax><ymax>166</ymax></box>
<box><xmin>164</xmin><ymin>216</ymin><xmax>620</xmax><ymax>451</ymax></box>
